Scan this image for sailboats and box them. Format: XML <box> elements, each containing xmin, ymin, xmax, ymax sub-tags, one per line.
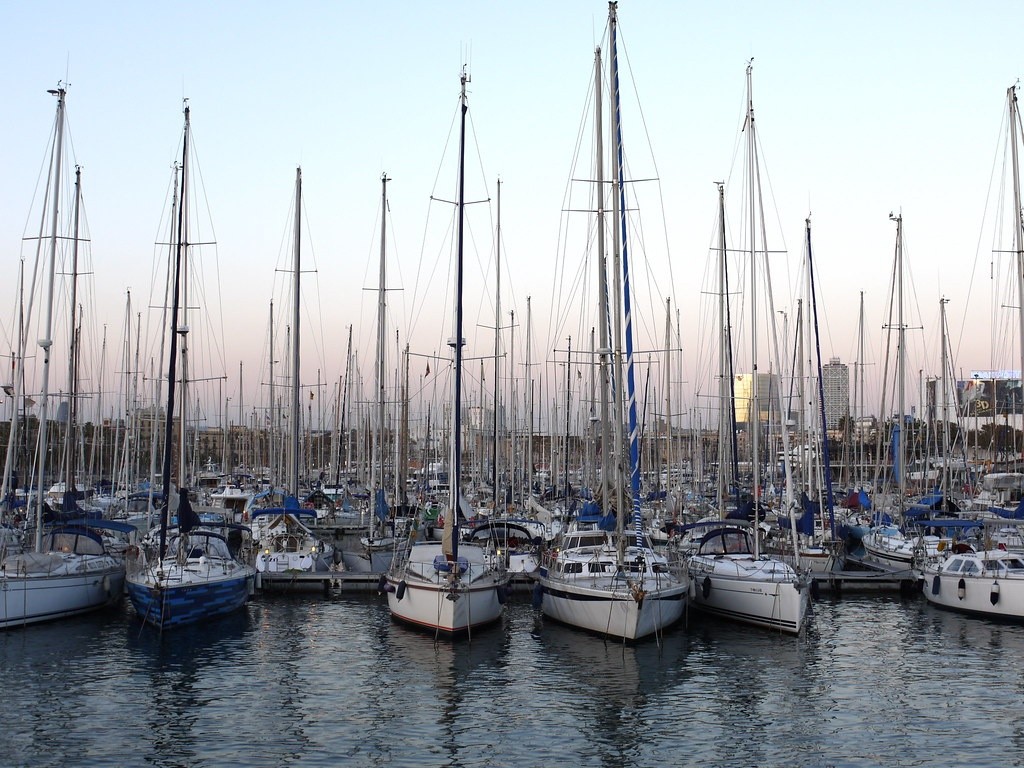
<box><xmin>921</xmin><ymin>85</ymin><xmax>1024</xmax><ymax>623</ymax></box>
<box><xmin>688</xmin><ymin>64</ymin><xmax>814</xmax><ymax>634</ymax></box>
<box><xmin>0</xmin><ymin>165</ymin><xmax>1024</xmax><ymax>567</ymax></box>
<box><xmin>529</xmin><ymin>1</ymin><xmax>697</xmax><ymax>642</ymax></box>
<box><xmin>0</xmin><ymin>90</ymin><xmax>138</xmax><ymax>629</ymax></box>
<box><xmin>125</xmin><ymin>108</ymin><xmax>259</xmax><ymax>630</ymax></box>
<box><xmin>385</xmin><ymin>71</ymin><xmax>513</xmax><ymax>639</ymax></box>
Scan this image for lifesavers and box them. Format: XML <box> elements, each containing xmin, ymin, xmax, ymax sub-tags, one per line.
<box><xmin>304</xmin><ymin>501</ymin><xmax>314</xmax><ymax>510</ymax></box>
